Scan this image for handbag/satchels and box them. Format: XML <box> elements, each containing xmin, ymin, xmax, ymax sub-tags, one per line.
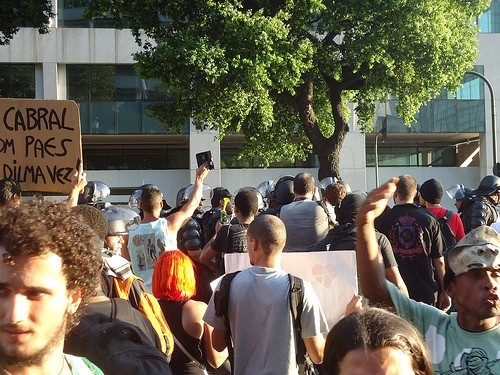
<box><xmin>185</xmin><ymin>357</ymin><xmax>208</xmax><ymax>375</ymax></box>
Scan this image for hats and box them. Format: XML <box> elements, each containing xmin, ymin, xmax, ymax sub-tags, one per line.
<box><xmin>468</xmin><ymin>176</ymin><xmax>500</xmax><ymax>196</ymax></box>
<box><xmin>449</xmin><ymin>225</ymin><xmax>500</xmax><ymax>275</ymax></box>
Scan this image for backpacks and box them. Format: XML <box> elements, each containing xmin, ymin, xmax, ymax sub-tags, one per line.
<box><xmin>221</xmin><ymin>271</ymin><xmax>309</xmax><ymax>368</ymax></box>
<box><xmin>78</xmin><ymin>298</ymin><xmax>172</xmax><ymax>375</ymax></box>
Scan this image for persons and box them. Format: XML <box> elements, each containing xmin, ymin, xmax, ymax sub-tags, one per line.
<box><xmin>152</xmin><ymin>250</ymin><xmax>229</xmax><ymax>375</ymax></box>
<box><xmin>0</xmin><ymin>162</ymin><xmax>500</xmax><ymax>313</ymax></box>
<box><xmin>202</xmin><ymin>214</ymin><xmax>330</xmax><ymax>375</ymax></box>
<box><xmin>319</xmin><ymin>306</ymin><xmax>434</xmax><ymax>375</ymax></box>
<box><xmin>70</xmin><ymin>205</ymin><xmax>171</xmax><ymax>363</ymax></box>
<box><xmin>0</xmin><ymin>199</ymin><xmax>105</xmax><ymax>375</ymax></box>
<box><xmin>64</xmin><ymin>229</ymin><xmax>167</xmax><ymax>375</ymax></box>
<box><xmin>356</xmin><ymin>177</ymin><xmax>500</xmax><ymax>375</ymax></box>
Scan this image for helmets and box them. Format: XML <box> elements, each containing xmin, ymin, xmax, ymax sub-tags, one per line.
<box><xmin>79</xmin><ymin>175</ymin><xmax>500</xmax><ymax>235</ymax></box>
